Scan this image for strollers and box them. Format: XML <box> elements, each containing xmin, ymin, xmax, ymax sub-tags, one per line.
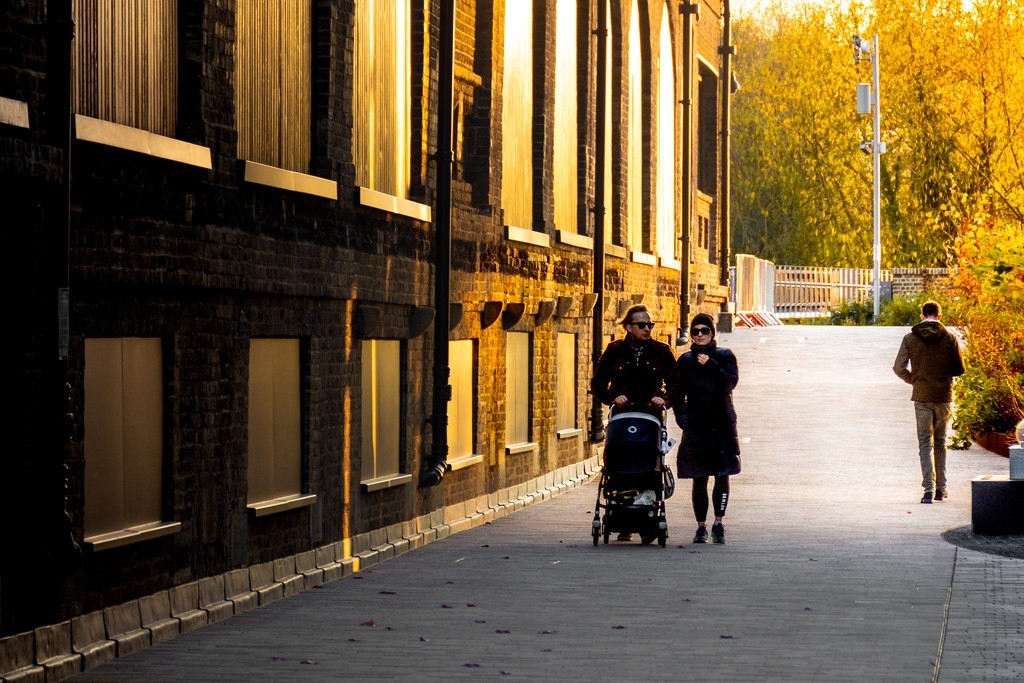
<box><xmin>591</xmin><ymin>400</ymin><xmax>676</xmax><ymax>549</ymax></box>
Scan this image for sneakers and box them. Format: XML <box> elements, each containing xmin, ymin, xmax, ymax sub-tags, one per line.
<box><xmin>934</xmin><ymin>490</ymin><xmax>948</xmax><ymax>500</ymax></box>
<box><xmin>920</xmin><ymin>493</ymin><xmax>933</xmax><ymax>504</ymax></box>
<box><xmin>711</xmin><ymin>523</ymin><xmax>727</xmax><ymax>544</ymax></box>
<box><xmin>693</xmin><ymin>525</ymin><xmax>708</xmax><ymax>543</ymax></box>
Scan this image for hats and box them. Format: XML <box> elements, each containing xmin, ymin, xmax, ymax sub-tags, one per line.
<box><xmin>689</xmin><ymin>312</ymin><xmax>716</xmax><ymax>340</ymax></box>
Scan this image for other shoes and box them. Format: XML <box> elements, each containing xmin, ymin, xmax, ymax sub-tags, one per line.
<box><xmin>616</xmin><ymin>533</ymin><xmax>635</xmax><ymax>542</ymax></box>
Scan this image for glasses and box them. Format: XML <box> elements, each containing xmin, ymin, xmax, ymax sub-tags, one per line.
<box><xmin>690</xmin><ymin>327</ymin><xmax>711</xmax><ymax>337</ymax></box>
<box><xmin>629</xmin><ymin>321</ymin><xmax>655</xmax><ymax>329</ymax></box>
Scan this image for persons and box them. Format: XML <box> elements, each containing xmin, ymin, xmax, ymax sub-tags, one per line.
<box><xmin>893</xmin><ymin>300</ymin><xmax>966</xmax><ymax>504</ymax></box>
<box><xmin>590</xmin><ymin>304</ymin><xmax>676</xmax><ymax>544</ymax></box>
<box><xmin>670</xmin><ymin>312</ymin><xmax>742</xmax><ymax>544</ymax></box>
